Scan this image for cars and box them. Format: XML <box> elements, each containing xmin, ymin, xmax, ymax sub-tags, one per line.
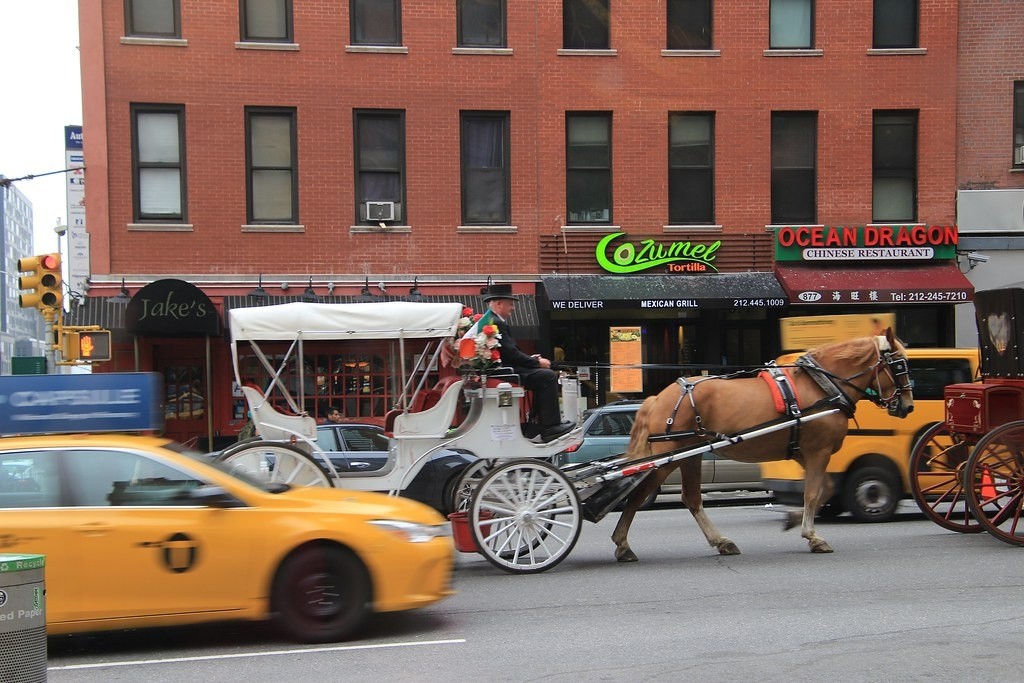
<box><xmin>559</xmin><ymin>401</ymin><xmax>771</xmax><ymax>495</ymax></box>
<box><xmin>0</xmin><ymin>427</ymin><xmax>459</xmax><ymax>646</ymax></box>
<box><xmin>293</xmin><ymin>419</ymin><xmax>396</xmax><ymax>474</ymax></box>
<box><xmin>760</xmin><ymin>350</ymin><xmax>1011</xmax><ymax>523</ymax></box>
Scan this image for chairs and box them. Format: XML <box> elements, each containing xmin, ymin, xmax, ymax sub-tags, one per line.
<box><xmin>462</xmin><ymin>339</ymin><xmax>524</xmax><ymax>396</ymax></box>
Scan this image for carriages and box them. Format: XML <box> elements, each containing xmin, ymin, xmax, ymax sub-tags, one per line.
<box><xmin>224</xmin><ymin>297</ymin><xmax>914</xmax><ymax>573</ymax></box>
<box><xmin>909</xmin><ymin>380</ymin><xmax>1024</xmax><ymax>551</ymax></box>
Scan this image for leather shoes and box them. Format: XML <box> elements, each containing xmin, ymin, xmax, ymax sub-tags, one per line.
<box><xmin>540</xmin><ymin>420</ymin><xmax>576</xmax><ymax>443</ymax></box>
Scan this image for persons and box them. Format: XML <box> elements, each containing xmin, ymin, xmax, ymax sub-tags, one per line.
<box><xmin>322</xmin><ymin>407</ymin><xmax>340</xmax><ymax>425</ymax></box>
<box><xmin>717</xmin><ymin>335</ymin><xmax>784</xmax><ymax>375</ymax></box>
<box><xmin>477</xmin><ymin>284</ymin><xmax>577</xmax><ymax>443</ymax></box>
<box><xmin>535</xmin><ymin>337</ymin><xmax>587</xmax><ymax>372</ymax></box>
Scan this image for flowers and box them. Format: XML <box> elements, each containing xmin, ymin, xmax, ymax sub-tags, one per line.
<box><xmin>449</xmin><ymin>307</ymin><xmax>502</xmax><ymax>388</ymax></box>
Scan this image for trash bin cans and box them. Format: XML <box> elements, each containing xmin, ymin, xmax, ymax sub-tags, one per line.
<box><xmin>0</xmin><ymin>553</ymin><xmax>50</xmax><ymax>683</ymax></box>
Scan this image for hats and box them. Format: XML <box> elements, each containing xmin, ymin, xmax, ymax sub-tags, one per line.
<box><xmin>483</xmin><ymin>284</ymin><xmax>520</xmax><ymax>303</ymax></box>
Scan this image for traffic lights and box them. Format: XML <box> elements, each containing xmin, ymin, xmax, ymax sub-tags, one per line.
<box><xmin>17</xmin><ymin>258</ymin><xmax>41</xmax><ymax>309</ymax></box>
<box><xmin>77</xmin><ymin>331</ymin><xmax>112</xmax><ymax>364</ymax></box>
<box><xmin>41</xmin><ymin>252</ymin><xmax>67</xmax><ymax>312</ymax></box>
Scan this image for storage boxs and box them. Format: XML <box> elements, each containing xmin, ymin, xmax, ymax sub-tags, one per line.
<box><xmin>944</xmin><ymin>383</ymin><xmax>1024</xmax><ymax>436</ymax></box>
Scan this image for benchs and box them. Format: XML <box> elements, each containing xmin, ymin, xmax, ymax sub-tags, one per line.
<box><xmin>394</xmin><ymin>379</ymin><xmax>463</xmax><ymax>465</ymax></box>
<box><xmin>243</xmin><ymin>386</ymin><xmax>317</xmax><ymax>457</ymax></box>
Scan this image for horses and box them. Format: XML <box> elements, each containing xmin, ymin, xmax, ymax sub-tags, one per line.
<box><xmin>609</xmin><ymin>326</ymin><xmax>915</xmax><ymax>563</ymax></box>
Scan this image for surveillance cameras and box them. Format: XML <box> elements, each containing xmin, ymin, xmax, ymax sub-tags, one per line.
<box><xmin>967</xmin><ymin>253</ymin><xmax>990</xmax><ymax>262</ymax></box>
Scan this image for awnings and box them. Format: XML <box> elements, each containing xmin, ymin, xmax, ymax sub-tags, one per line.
<box><xmin>775</xmin><ymin>265</ymin><xmax>975</xmax><ymax>304</ymax></box>
<box><xmin>61</xmin><ymin>296</ymin><xmax>135</xmax><ymax>349</ymax></box>
<box><xmin>540</xmin><ymin>273</ymin><xmax>788</xmax><ymax>312</ymax></box>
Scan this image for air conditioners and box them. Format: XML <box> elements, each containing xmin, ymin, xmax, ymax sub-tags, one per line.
<box><xmin>366</xmin><ymin>201</ymin><xmax>394</xmax><ymax>221</ymax></box>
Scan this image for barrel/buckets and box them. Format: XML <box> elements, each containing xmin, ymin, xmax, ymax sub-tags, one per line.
<box><xmin>447</xmin><ymin>510</ymin><xmax>494</xmax><ymax>553</ymax></box>
